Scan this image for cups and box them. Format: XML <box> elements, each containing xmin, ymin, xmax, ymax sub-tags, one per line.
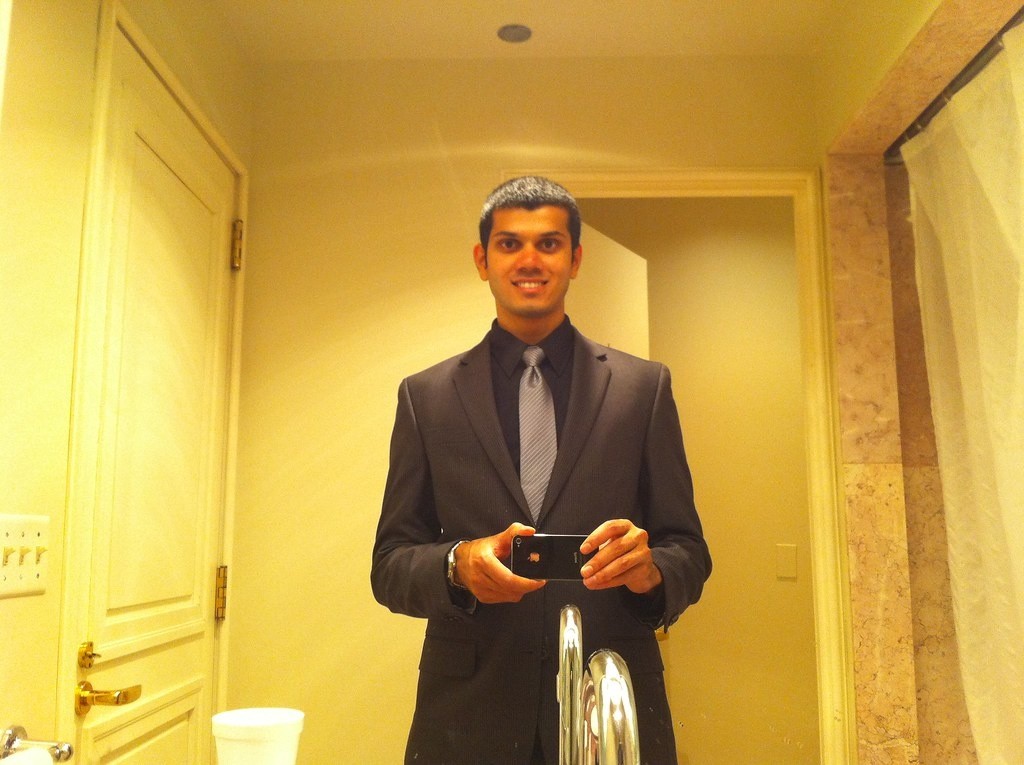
<box><xmin>211</xmin><ymin>708</ymin><xmax>305</xmax><ymax>765</ymax></box>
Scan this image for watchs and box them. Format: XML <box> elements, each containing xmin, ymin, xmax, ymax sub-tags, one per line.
<box><xmin>445</xmin><ymin>540</ymin><xmax>471</xmax><ymax>591</ymax></box>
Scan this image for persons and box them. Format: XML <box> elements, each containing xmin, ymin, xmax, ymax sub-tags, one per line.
<box><xmin>370</xmin><ymin>176</ymin><xmax>713</xmax><ymax>765</ymax></box>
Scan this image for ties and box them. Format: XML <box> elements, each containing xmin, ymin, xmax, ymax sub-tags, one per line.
<box><xmin>518</xmin><ymin>345</ymin><xmax>558</xmax><ymax>527</ymax></box>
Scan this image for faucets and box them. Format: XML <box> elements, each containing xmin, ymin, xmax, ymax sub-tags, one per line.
<box><xmin>555</xmin><ymin>604</ymin><xmax>584</xmax><ymax>765</ymax></box>
<box><xmin>577</xmin><ymin>647</ymin><xmax>640</xmax><ymax>765</ymax></box>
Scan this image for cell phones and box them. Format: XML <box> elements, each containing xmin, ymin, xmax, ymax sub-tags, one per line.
<box><xmin>511</xmin><ymin>532</ymin><xmax>598</xmax><ymax>583</ymax></box>
